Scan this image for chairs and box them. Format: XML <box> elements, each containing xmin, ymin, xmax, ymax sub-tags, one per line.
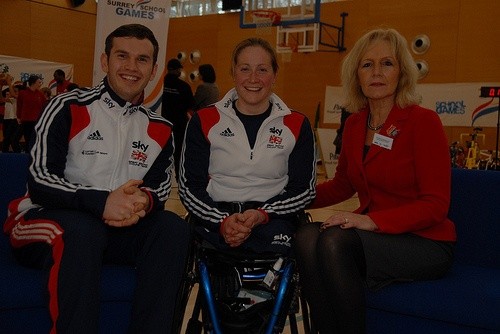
<box><xmin>183</xmin><ymin>213</ymin><xmax>314</xmax><ymax>334</ymax></box>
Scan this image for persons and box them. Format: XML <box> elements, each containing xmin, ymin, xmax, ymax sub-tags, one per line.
<box><xmin>48</xmin><ymin>69</ymin><xmax>72</xmax><ymax>96</ymax></box>
<box><xmin>1</xmin><ymin>22</ymin><xmax>189</xmax><ymax>334</ymax></box>
<box><xmin>2</xmin><ymin>81</ymin><xmax>23</xmax><ymax>154</ymax></box>
<box><xmin>66</xmin><ymin>82</ymin><xmax>79</xmax><ymax>92</ymax></box>
<box><xmin>178</xmin><ymin>37</ymin><xmax>318</xmax><ymax>334</ymax></box>
<box><xmin>192</xmin><ymin>64</ymin><xmax>221</xmax><ymax>114</ymax></box>
<box><xmin>4</xmin><ymin>75</ymin><xmax>48</xmax><ymax>152</ymax></box>
<box><xmin>160</xmin><ymin>58</ymin><xmax>194</xmax><ymax>183</ymax></box>
<box><xmin>306</xmin><ymin>27</ymin><xmax>457</xmax><ymax>334</ymax></box>
<box><xmin>40</xmin><ymin>87</ymin><xmax>53</xmax><ymax>99</ymax></box>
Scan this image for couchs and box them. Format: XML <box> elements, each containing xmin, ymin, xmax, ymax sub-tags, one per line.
<box><xmin>0</xmin><ymin>152</ymin><xmax>138</xmax><ymax>334</ymax></box>
<box><xmin>297</xmin><ymin>168</ymin><xmax>500</xmax><ymax>334</ymax></box>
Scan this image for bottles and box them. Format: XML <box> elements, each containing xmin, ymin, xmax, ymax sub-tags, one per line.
<box><xmin>263</xmin><ymin>257</ymin><xmax>285</xmax><ymax>289</ymax></box>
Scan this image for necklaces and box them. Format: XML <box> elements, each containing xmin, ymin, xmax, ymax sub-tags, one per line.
<box><xmin>367</xmin><ymin>112</ymin><xmax>385</xmax><ymax>131</ymax></box>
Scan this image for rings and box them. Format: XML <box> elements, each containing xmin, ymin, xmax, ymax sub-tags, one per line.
<box><xmin>345</xmin><ymin>217</ymin><xmax>349</xmax><ymax>224</ymax></box>
